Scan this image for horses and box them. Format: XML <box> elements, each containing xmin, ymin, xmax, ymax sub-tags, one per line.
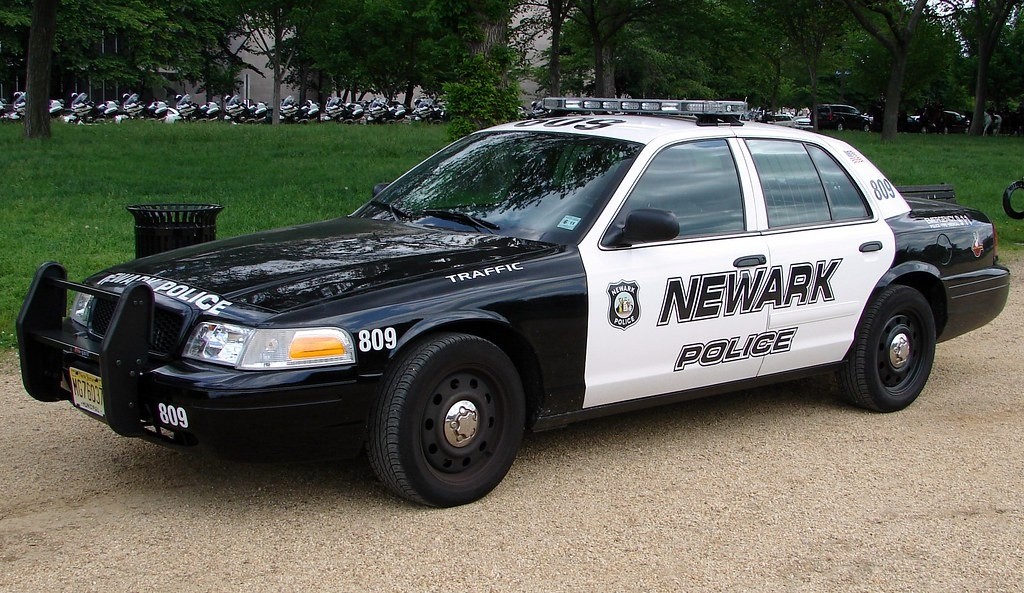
<box><xmin>923</xmin><ymin>105</ymin><xmax>945</xmax><ymax>134</ymax></box>
<box><xmin>965</xmin><ymin>112</ymin><xmax>974</xmax><ymax>132</ymax></box>
<box><xmin>983</xmin><ymin>111</ymin><xmax>1002</xmax><ymax>136</ymax></box>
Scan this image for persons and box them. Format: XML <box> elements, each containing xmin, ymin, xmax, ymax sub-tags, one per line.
<box><xmin>869</xmin><ymin>92</ymin><xmax>1024</xmax><ymax>137</ymax></box>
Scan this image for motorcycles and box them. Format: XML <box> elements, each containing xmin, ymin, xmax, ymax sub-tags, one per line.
<box><xmin>0</xmin><ymin>88</ymin><xmax>454</xmax><ymax>127</ymax></box>
<box><xmin>516</xmin><ymin>99</ymin><xmax>552</xmax><ymax>122</ymax></box>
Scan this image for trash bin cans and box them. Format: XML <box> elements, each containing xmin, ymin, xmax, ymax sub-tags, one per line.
<box><xmin>123</xmin><ymin>201</ymin><xmax>226</xmax><ymax>261</ymax></box>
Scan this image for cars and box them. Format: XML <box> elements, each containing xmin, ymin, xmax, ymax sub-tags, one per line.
<box><xmin>809</xmin><ymin>102</ymin><xmax>874</xmax><ymax>133</ymax></box>
<box><xmin>13</xmin><ymin>98</ymin><xmax>1012</xmax><ymax>511</ymax></box>
<box><xmin>904</xmin><ymin>110</ymin><xmax>971</xmax><ymax>135</ymax></box>
<box><xmin>745</xmin><ymin>109</ymin><xmax>814</xmax><ymax>131</ymax></box>
